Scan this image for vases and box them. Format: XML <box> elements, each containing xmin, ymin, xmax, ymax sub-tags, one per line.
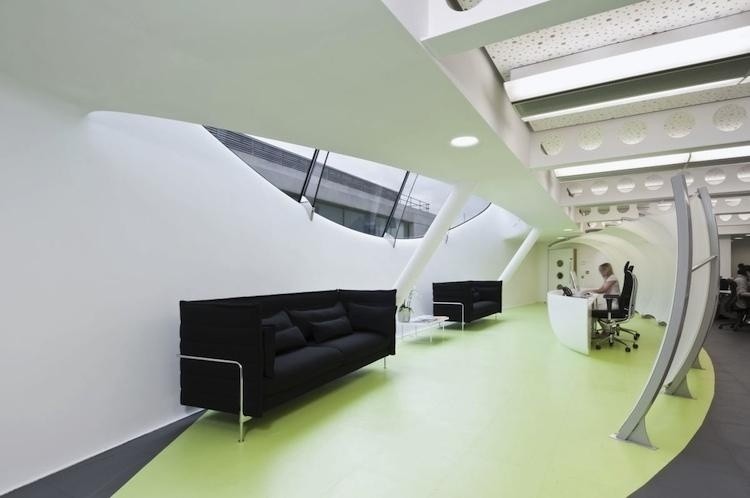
<box><xmin>397</xmin><ymin>308</ymin><xmax>415</xmax><ymax>323</ymax></box>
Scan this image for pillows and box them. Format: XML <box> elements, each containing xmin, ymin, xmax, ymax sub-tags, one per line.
<box><xmin>259</xmin><ymin>304</ymin><xmax>354</xmax><ymax>355</ymax></box>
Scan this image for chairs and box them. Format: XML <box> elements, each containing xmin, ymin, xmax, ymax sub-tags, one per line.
<box><xmin>591</xmin><ymin>261</ymin><xmax>640</xmax><ymax>352</ymax></box>
<box><xmin>715</xmin><ymin>275</ymin><xmax>750</xmax><ymax>331</ymax></box>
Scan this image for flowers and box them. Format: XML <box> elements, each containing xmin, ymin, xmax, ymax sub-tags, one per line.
<box><xmin>397</xmin><ymin>287</ymin><xmax>416</xmax><ymax>307</ymax></box>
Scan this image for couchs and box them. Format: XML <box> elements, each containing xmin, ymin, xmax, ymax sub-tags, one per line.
<box><xmin>179</xmin><ymin>288</ymin><xmax>397</xmax><ymax>441</ymax></box>
<box><xmin>432</xmin><ymin>281</ymin><xmax>503</xmax><ymax>331</ymax></box>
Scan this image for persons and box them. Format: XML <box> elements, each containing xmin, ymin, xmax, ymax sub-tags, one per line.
<box><xmin>580</xmin><ymin>263</ymin><xmax>622</xmax><ymax>338</ymax></box>
<box><xmin>728</xmin><ymin>263</ymin><xmax>750</xmax><ymax>324</ymax></box>
